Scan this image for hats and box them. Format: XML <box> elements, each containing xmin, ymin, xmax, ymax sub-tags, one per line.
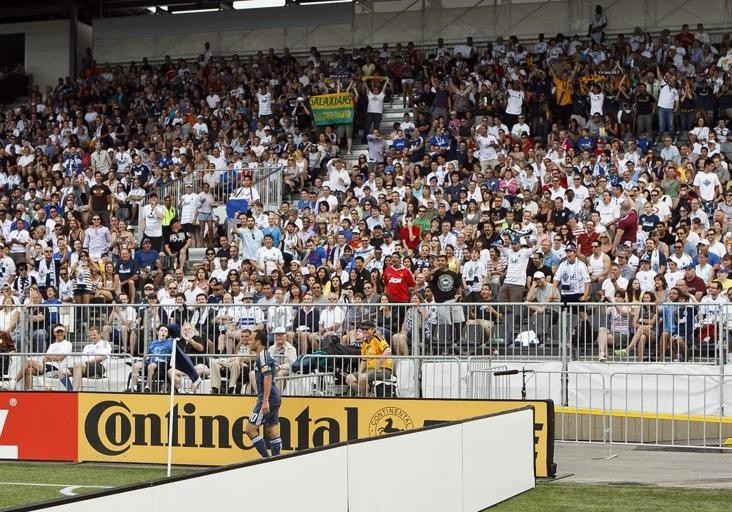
<box><xmin>48</xmin><ymin>96</ymin><xmax>717</xmax><ymax>266</ymax></box>
<box><xmin>289</xmin><ymin>259</ymin><xmax>300</xmax><ymax>265</ymax></box>
<box><xmin>241</xmin><ymin>291</ymin><xmax>254</xmax><ymax>301</ymax></box>
<box><xmin>533</xmin><ymin>271</ymin><xmax>545</xmax><ymax>280</ymax></box>
<box><xmin>54</xmin><ymin>325</ymin><xmax>66</xmax><ymax>332</ymax></box>
<box><xmin>269</xmin><ymin>325</ymin><xmax>286</xmax><ymax>335</ymax></box>
<box><xmin>357</xmin><ymin>320</ymin><xmax>377</xmax><ymax>329</ymax></box>
<box><xmin>144</xmin><ymin>283</ymin><xmax>155</xmax><ymax>289</ymax></box>
<box><xmin>714</xmin><ymin>265</ymin><xmax>729</xmax><ymax>274</ymax></box>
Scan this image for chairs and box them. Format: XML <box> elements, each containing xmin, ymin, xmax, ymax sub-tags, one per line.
<box><xmin>427</xmin><ymin>311</ymin><xmax>611</xmax><ymax>355</ymax></box>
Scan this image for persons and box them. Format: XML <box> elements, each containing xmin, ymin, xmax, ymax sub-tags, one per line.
<box><xmin>244</xmin><ymin>329</ymin><xmax>281</xmax><ymax>457</ymax></box>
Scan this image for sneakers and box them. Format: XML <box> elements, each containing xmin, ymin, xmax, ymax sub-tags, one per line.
<box><xmin>0</xmin><ymin>380</ymin><xmax>17</xmax><ymax>391</ymax></box>
<box><xmin>614</xmin><ymin>348</ymin><xmax>630</xmax><ymax>357</ymax></box>
<box><xmin>123</xmin><ymin>387</ymin><xmax>151</xmax><ymax>394</ymax></box>
<box><xmin>598</xmin><ymin>352</ymin><xmax>606</xmax><ymax>362</ymax></box>
